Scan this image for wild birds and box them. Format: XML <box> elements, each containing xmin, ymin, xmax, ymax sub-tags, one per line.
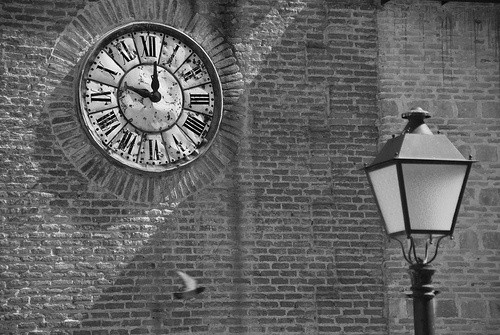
<box><xmin>173</xmin><ymin>270</ymin><xmax>208</xmax><ymax>301</ymax></box>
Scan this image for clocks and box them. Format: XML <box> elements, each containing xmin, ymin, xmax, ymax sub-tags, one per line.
<box><xmin>77</xmin><ymin>22</ymin><xmax>224</xmax><ymax>178</ymax></box>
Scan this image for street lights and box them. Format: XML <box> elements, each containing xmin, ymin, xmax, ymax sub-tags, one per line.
<box><xmin>359</xmin><ymin>108</ymin><xmax>478</xmax><ymax>335</ymax></box>
